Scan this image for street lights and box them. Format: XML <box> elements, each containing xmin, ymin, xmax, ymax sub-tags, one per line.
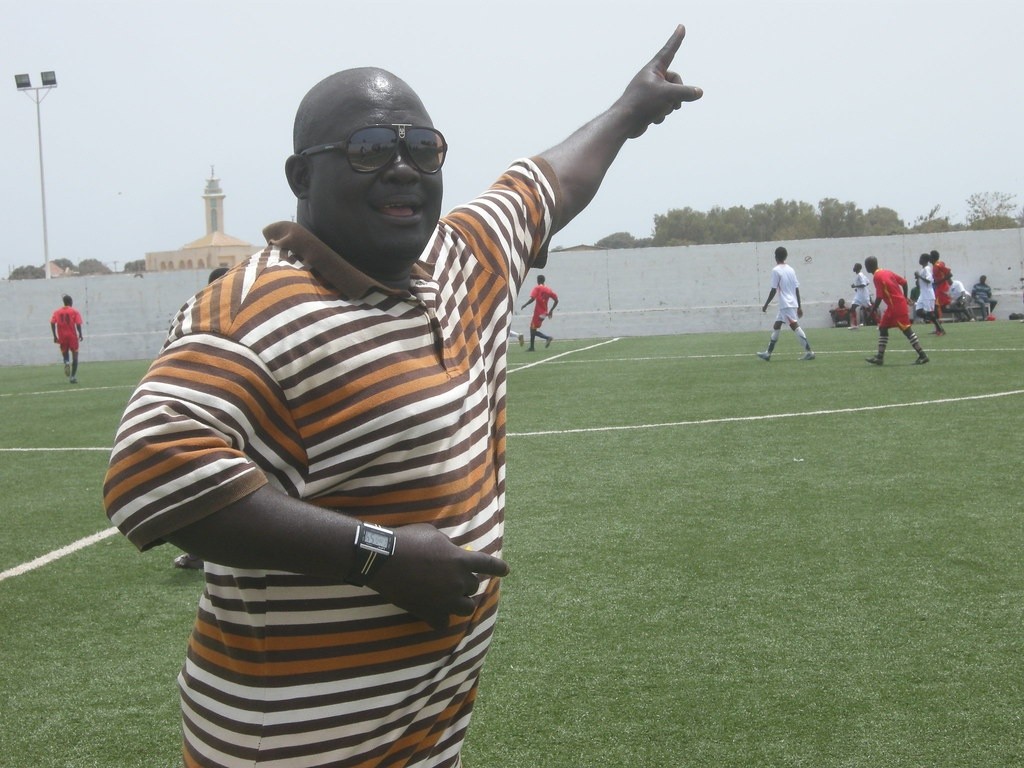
<box><xmin>13</xmin><ymin>69</ymin><xmax>58</xmax><ymax>281</ymax></box>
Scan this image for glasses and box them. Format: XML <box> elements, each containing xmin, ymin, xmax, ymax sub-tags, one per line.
<box><xmin>299</xmin><ymin>123</ymin><xmax>449</xmax><ymax>175</ymax></box>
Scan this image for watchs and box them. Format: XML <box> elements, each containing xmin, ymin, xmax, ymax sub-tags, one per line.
<box><xmin>346</xmin><ymin>512</ymin><xmax>397</xmax><ymax>585</ymax></box>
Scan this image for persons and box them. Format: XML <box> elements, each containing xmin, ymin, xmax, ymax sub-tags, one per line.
<box><xmin>51</xmin><ymin>296</ymin><xmax>83</xmax><ymax>383</ymax></box>
<box><xmin>755</xmin><ymin>247</ymin><xmax>815</xmax><ymax>361</ymax></box>
<box><xmin>173</xmin><ymin>267</ymin><xmax>230</xmax><ymax>569</ymax></box>
<box><xmin>915</xmin><ymin>254</ymin><xmax>945</xmax><ymax>336</ymax></box>
<box><xmin>829</xmin><ymin>298</ymin><xmax>852</xmax><ymax>327</ymax></box>
<box><xmin>972</xmin><ymin>275</ymin><xmax>998</xmax><ymax>321</ymax></box>
<box><xmin>102</xmin><ymin>25</ymin><xmax>706</xmax><ymax>768</ymax></box>
<box><xmin>521</xmin><ymin>275</ymin><xmax>559</xmax><ymax>352</ymax></box>
<box><xmin>848</xmin><ymin>264</ymin><xmax>881</xmax><ymax>330</ymax></box>
<box><xmin>863</xmin><ymin>255</ymin><xmax>928</xmax><ymax>365</ymax></box>
<box><xmin>949</xmin><ymin>278</ymin><xmax>974</xmax><ymax>322</ymax></box>
<box><xmin>911</xmin><ymin>280</ymin><xmax>920</xmax><ymax>323</ymax></box>
<box><xmin>929</xmin><ymin>250</ymin><xmax>953</xmax><ymax>320</ymax></box>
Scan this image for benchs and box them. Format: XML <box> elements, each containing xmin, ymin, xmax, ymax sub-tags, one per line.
<box><xmin>937</xmin><ymin>303</ymin><xmax>990</xmax><ymax>322</ymax></box>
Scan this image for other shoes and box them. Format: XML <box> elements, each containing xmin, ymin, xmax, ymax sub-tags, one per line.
<box><xmin>545</xmin><ymin>336</ymin><xmax>553</xmax><ymax>348</ymax></box>
<box><xmin>970</xmin><ymin>317</ymin><xmax>976</xmax><ymax>323</ymax></box>
<box><xmin>847</xmin><ymin>325</ymin><xmax>858</xmax><ymax>329</ymax></box>
<box><xmin>69</xmin><ymin>374</ymin><xmax>77</xmax><ymax>383</ymax></box>
<box><xmin>175</xmin><ymin>552</ymin><xmax>206</xmax><ymax>571</ymax></box>
<box><xmin>936</xmin><ymin>331</ymin><xmax>945</xmax><ymax>337</ymax></box>
<box><xmin>756</xmin><ymin>351</ymin><xmax>770</xmax><ymax>362</ymax></box>
<box><xmin>525</xmin><ymin>346</ymin><xmax>535</xmax><ymax>351</ymax></box>
<box><xmin>798</xmin><ymin>351</ymin><xmax>816</xmax><ymax>360</ymax></box>
<box><xmin>913</xmin><ymin>354</ymin><xmax>929</xmax><ymax>366</ymax></box>
<box><xmin>518</xmin><ymin>333</ymin><xmax>524</xmax><ymax>346</ymax></box>
<box><xmin>64</xmin><ymin>361</ymin><xmax>72</xmax><ymax>377</ymax></box>
<box><xmin>865</xmin><ymin>355</ymin><xmax>886</xmax><ymax>366</ymax></box>
